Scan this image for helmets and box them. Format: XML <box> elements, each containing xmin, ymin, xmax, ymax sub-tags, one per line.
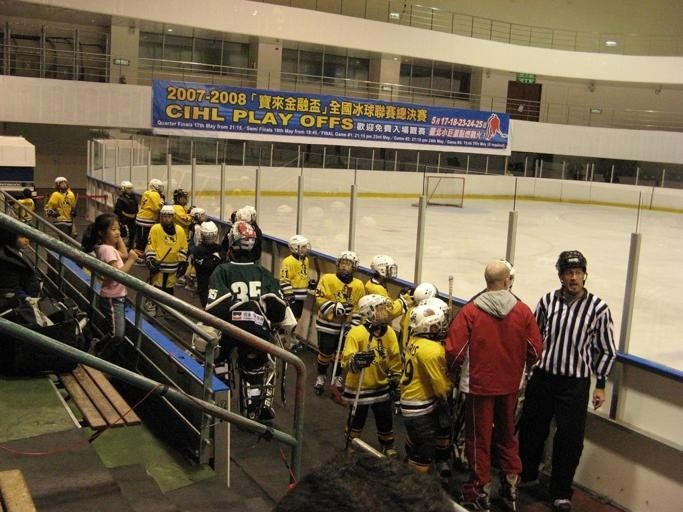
<box><xmin>117</xmin><ymin>179</ymin><xmax>311</xmax><ymax>257</ymax></box>
<box><xmin>55</xmin><ymin>177</ymin><xmax>68</xmax><ymax>192</ymax></box>
<box><xmin>330</xmin><ymin>250</ymin><xmax>589</xmax><ymax>338</ymax></box>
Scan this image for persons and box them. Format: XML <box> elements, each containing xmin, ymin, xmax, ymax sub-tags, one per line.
<box><xmin>315</xmin><ymin>250</ymin><xmax>455</xmax><ymax>482</ymax></box>
<box><xmin>0</xmin><ymin>224</ymin><xmax>41</xmax><ymax>297</ymax></box>
<box><xmin>13</xmin><ymin>175</ymin><xmax>76</xmax><ymax>243</ymax></box>
<box><xmin>81</xmin><ymin>212</ymin><xmax>138</xmax><ymax>339</ymax></box>
<box><xmin>114</xmin><ymin>178</ymin><xmax>196</xmax><ymax>318</ymax></box>
<box><xmin>518</xmin><ymin>250</ymin><xmax>616</xmax><ymax>510</ymax></box>
<box><xmin>446</xmin><ymin>259</ymin><xmax>542</xmax><ymax>512</ymax></box>
<box><xmin>185</xmin><ymin>206</ymin><xmax>315</xmax><ymax>425</ymax></box>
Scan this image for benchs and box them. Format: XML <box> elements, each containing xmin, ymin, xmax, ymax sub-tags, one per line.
<box><xmin>47</xmin><ymin>340</ymin><xmax>142</xmax><ymax>434</ymax></box>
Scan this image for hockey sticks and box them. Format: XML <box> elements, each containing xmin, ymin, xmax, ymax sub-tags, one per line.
<box><xmin>330</xmin><ymin>318</ymin><xmax>345</xmax><ymax>401</ymax></box>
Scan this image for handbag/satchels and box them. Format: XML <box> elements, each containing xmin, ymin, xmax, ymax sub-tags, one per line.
<box><xmin>0</xmin><ymin>291</ymin><xmax>92</xmax><ymax>376</ymax></box>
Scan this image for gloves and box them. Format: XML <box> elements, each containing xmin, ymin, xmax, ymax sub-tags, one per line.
<box><xmin>175</xmin><ymin>262</ymin><xmax>189</xmax><ymax>277</ymax></box>
<box><xmin>355</xmin><ymin>350</ymin><xmax>375</xmax><ymax>368</ymax></box>
<box><xmin>146</xmin><ymin>258</ymin><xmax>159</xmax><ymax>273</ymax></box>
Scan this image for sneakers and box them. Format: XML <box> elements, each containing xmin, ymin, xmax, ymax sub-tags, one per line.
<box><xmin>130</xmin><ymin>277</ymin><xmax>306</xmax><ymax>352</ymax></box>
<box><xmin>314</xmin><ymin>374</ymin><xmax>578</xmax><ymax>511</ymax></box>
